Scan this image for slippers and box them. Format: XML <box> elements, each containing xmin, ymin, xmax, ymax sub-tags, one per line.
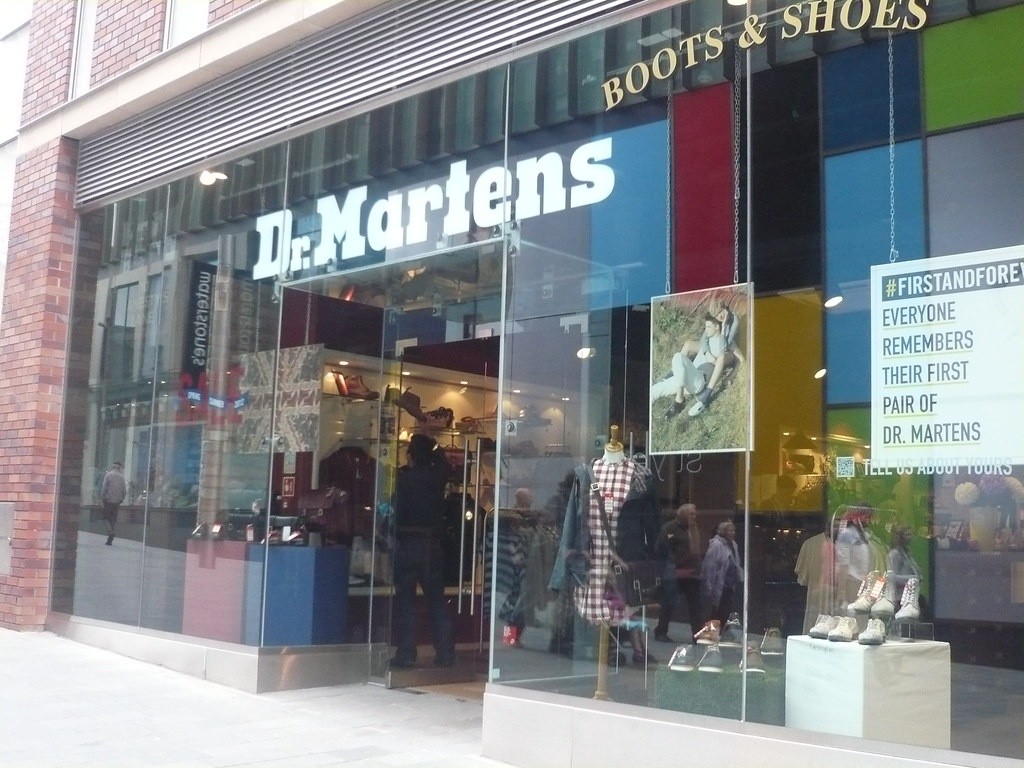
<box><xmin>456</xmin><ymin>417</ymin><xmax>476</xmax><ymax>427</ymax></box>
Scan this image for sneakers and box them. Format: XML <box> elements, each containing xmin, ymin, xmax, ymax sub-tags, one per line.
<box><xmin>808</xmin><ymin>613</ymin><xmax>833</xmax><ymax>639</ymax></box>
<box><xmin>689</xmin><ymin>400</ymin><xmax>705</xmax><ymax>417</ymax></box>
<box><xmin>665</xmin><ymin>397</ymin><xmax>686</xmax><ymax>419</ymax></box>
<box><xmin>828</xmin><ymin>617</ymin><xmax>859</xmax><ymax>642</ymax></box>
<box><xmin>425</xmin><ymin>406</ymin><xmax>454</xmax><ymax>425</ymax></box>
<box><xmin>859</xmin><ymin>618</ymin><xmax>885</xmax><ymax>645</ymax></box>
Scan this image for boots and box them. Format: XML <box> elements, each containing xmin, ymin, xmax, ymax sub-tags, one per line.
<box><xmin>854</xmin><ymin>570</ymin><xmax>880</xmax><ymax>613</ymax></box>
<box><xmin>894</xmin><ymin>577</ymin><xmax>920</xmax><ymax>620</ymax></box>
<box><xmin>871</xmin><ymin>570</ymin><xmax>895</xmax><ymax>615</ymax></box>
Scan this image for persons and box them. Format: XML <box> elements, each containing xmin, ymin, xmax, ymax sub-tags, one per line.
<box><xmin>885</xmin><ymin>522</ymin><xmax>923</xmax><ymax>614</ymax></box>
<box><xmin>511</xmin><ymin>488</ymin><xmax>744</xmax><ymax>670</ymax></box>
<box><xmin>650</xmin><ymin>301</ymin><xmax>744</xmax><ymax>420</ymax></box>
<box><xmin>100</xmin><ymin>461</ymin><xmax>126</xmax><ymax>546</ymax></box>
<box><xmin>761</xmin><ymin>474</ymin><xmax>797</xmax><ymax>512</ymax></box>
<box><xmin>392</xmin><ymin>433</ymin><xmax>457</xmax><ymax>667</ymax></box>
<box><xmin>547</xmin><ymin>441</ymin><xmax>659</xmax><ymax>627</ymax></box>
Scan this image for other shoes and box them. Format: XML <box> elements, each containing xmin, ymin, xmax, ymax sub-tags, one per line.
<box><xmin>694</xmin><ymin>619</ymin><xmax>720</xmax><ymax>645</ymax></box>
<box><xmin>633</xmin><ymin>650</ymin><xmax>658</xmax><ymax>664</ymax></box>
<box><xmin>738</xmin><ymin>641</ymin><xmax>765</xmax><ymax>673</ymax></box>
<box><xmin>656</xmin><ymin>635</ymin><xmax>674</xmax><ymax>642</ymax></box>
<box><xmin>438</xmin><ymin>658</ymin><xmax>454</xmax><ymax>667</ymax></box>
<box><xmin>668</xmin><ymin>644</ymin><xmax>695</xmax><ymax>671</ymax></box>
<box><xmin>718</xmin><ymin>611</ymin><xmax>743</xmax><ymax>647</ymax></box>
<box><xmin>402</xmin><ymin>388</ymin><xmax>426</xmax><ymax>422</ymax></box>
<box><xmin>476</xmin><ymin>437</ymin><xmax>496</xmax><ymax>453</ymax></box>
<box><xmin>345</xmin><ymin>375</ymin><xmax>379</xmax><ymax>399</ymax></box>
<box><xmin>761</xmin><ymin>627</ymin><xmax>783</xmax><ymax>656</ymax></box>
<box><xmin>696</xmin><ymin>643</ymin><xmax>723</xmax><ymax>673</ymax></box>
<box><xmin>387</xmin><ymin>657</ymin><xmax>415</xmax><ymax>669</ymax></box>
<box><xmin>105</xmin><ymin>532</ymin><xmax>114</xmax><ymax>545</ymax></box>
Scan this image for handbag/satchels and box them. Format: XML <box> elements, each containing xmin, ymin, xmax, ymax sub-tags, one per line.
<box><xmin>298</xmin><ymin>487</ymin><xmax>352</xmax><ymax>540</ymax></box>
<box><xmin>615</xmin><ymin>561</ymin><xmax>667</xmax><ymax>607</ymax></box>
<box><xmin>442</xmin><ymin>444</ymin><xmax>472</xmax><ymax>484</ymax></box>
